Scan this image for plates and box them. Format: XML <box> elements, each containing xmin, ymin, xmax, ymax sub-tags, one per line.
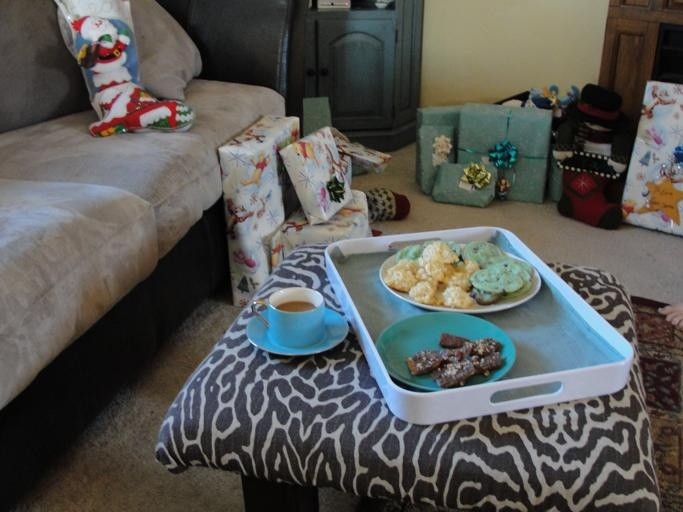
<box><xmin>245</xmin><ymin>309</ymin><xmax>350</xmax><ymax>357</ymax></box>
<box><xmin>375</xmin><ymin>242</ymin><xmax>542</xmax><ymax>392</ymax></box>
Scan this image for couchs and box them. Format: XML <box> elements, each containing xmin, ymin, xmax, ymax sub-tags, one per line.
<box><xmin>0</xmin><ymin>0</ymin><xmax>294</xmax><ymax>413</ymax></box>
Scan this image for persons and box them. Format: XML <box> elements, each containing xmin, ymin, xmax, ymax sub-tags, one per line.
<box><xmin>657</xmin><ymin>302</ymin><xmax>682</xmax><ymax>328</ymax></box>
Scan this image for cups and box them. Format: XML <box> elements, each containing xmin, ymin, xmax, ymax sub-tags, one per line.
<box><xmin>251</xmin><ymin>287</ymin><xmax>326</xmax><ymax>347</ymax></box>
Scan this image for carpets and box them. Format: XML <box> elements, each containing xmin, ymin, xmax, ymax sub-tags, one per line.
<box><xmin>353</xmin><ymin>293</ymin><xmax>683</xmax><ymax>512</ymax></box>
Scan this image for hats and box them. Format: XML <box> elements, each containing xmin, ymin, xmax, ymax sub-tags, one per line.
<box><xmin>566</xmin><ymin>83</ymin><xmax>631</xmax><ymax>129</ymax></box>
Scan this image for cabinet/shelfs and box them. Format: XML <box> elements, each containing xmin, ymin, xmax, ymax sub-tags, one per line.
<box><xmin>286</xmin><ymin>1</ymin><xmax>424</xmax><ymax>152</ymax></box>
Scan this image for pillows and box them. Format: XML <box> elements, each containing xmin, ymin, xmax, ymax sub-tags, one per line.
<box><xmin>127</xmin><ymin>0</ymin><xmax>205</xmax><ymax>101</ymax></box>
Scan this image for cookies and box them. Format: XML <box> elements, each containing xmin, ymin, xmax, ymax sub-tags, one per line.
<box><xmin>406</xmin><ymin>332</ymin><xmax>504</xmax><ymax>389</ymax></box>
<box><xmin>382</xmin><ymin>238</ymin><xmax>533</xmax><ymax>309</ymax></box>
